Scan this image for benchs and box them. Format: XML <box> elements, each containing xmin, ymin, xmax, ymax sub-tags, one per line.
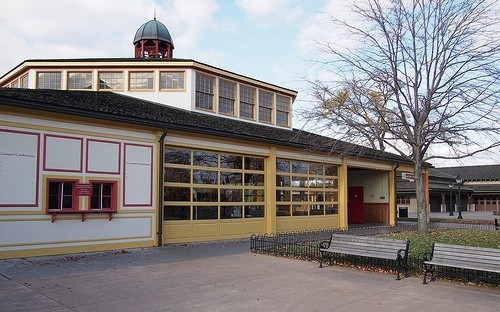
<box><xmin>494</xmin><ymin>218</ymin><xmax>500</xmax><ymax>231</ymax></box>
<box><xmin>421</xmin><ymin>243</ymin><xmax>500</xmax><ymax>290</ymax></box>
<box><xmin>317</xmin><ymin>233</ymin><xmax>409</xmax><ymax>282</ymax></box>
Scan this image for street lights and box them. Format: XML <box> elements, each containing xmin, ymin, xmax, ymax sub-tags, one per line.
<box><xmin>449</xmin><ymin>180</ymin><xmax>454</xmax><ymax>216</ymax></box>
<box><xmin>455</xmin><ymin>173</ymin><xmax>463</xmax><ymax>219</ymax></box>
